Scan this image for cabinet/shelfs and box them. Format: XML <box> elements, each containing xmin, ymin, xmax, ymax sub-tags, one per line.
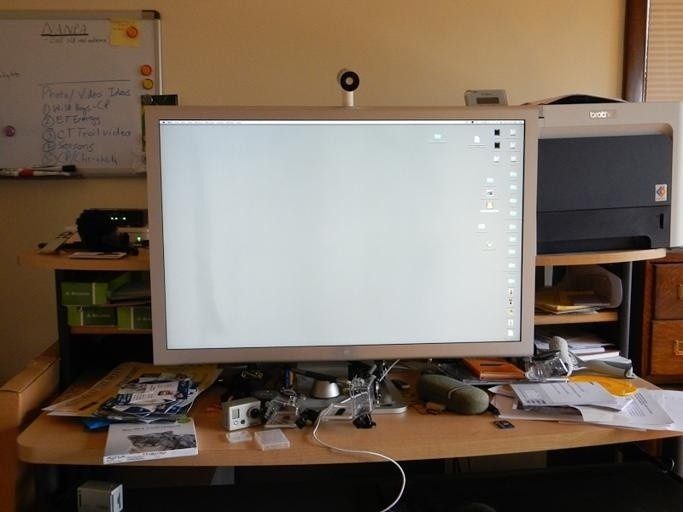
<box><xmin>13</xmin><ymin>227</ymin><xmax>668</xmax><ymax>366</ymax></box>
<box><xmin>628</xmin><ymin>249</ymin><xmax>682</xmax><ymax>456</ymax></box>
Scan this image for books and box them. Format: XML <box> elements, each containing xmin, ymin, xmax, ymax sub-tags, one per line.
<box><xmin>464</xmin><ymin>358</ymin><xmax>527</xmax><ymax>379</ymax></box>
<box><xmin>102</xmin><ymin>416</ymin><xmax>198</xmax><ymax>465</ymax></box>
<box><xmin>534</xmin><ymin>286</ymin><xmax>622</xmax><ymax>375</ymax></box>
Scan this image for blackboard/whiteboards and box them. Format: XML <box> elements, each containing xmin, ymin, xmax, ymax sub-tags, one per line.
<box><xmin>0</xmin><ymin>10</ymin><xmax>162</xmax><ymax>179</ymax></box>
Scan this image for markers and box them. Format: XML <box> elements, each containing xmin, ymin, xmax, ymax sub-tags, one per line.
<box><xmin>1</xmin><ymin>166</ymin><xmax>81</xmax><ymax>176</ymax></box>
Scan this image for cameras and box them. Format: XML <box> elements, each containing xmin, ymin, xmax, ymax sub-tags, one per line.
<box><xmin>221</xmin><ymin>396</ymin><xmax>262</xmax><ymax>431</ymax></box>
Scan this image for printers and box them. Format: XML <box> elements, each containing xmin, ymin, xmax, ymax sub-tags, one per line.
<box><xmin>518</xmin><ymin>94</ymin><xmax>683</xmax><ymax>256</ymax></box>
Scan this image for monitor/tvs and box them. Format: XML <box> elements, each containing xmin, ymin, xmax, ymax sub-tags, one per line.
<box><xmin>143</xmin><ymin>103</ymin><xmax>540</xmax><ymax>415</ymax></box>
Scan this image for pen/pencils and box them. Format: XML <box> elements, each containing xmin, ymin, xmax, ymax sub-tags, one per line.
<box><xmin>487</xmin><ymin>404</ymin><xmax>500</xmax><ymax>415</ymax></box>
<box><xmin>291</xmin><ymin>367</ymin><xmax>351</xmax><ymax>385</ymax></box>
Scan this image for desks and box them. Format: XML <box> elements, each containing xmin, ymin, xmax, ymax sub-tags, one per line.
<box><xmin>15</xmin><ymin>363</ymin><xmax>683</xmax><ymax>512</ymax></box>
